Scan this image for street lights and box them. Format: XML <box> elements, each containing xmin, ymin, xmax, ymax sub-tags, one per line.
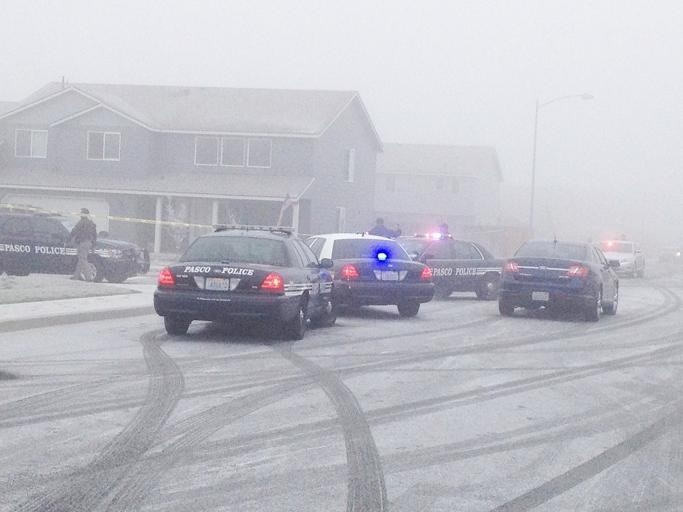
<box><xmin>526</xmin><ymin>91</ymin><xmax>595</xmax><ymax>232</ymax></box>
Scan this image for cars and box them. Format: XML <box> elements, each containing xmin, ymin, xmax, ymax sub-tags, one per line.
<box><xmin>303</xmin><ymin>230</ymin><xmax>436</xmax><ymax>317</ymax></box>
<box><xmin>154</xmin><ymin>224</ymin><xmax>339</xmax><ymax>342</ymax></box>
<box><xmin>601</xmin><ymin>239</ymin><xmax>646</xmax><ymax>278</ymax></box>
<box><xmin>394</xmin><ymin>234</ymin><xmax>503</xmax><ymax>301</ymax></box>
<box><xmin>2</xmin><ymin>211</ymin><xmax>151</xmax><ymax>283</ymax></box>
<box><xmin>497</xmin><ymin>237</ymin><xmax>620</xmax><ymax>324</ymax></box>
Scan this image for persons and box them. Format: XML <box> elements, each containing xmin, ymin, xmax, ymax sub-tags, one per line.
<box><xmin>438</xmin><ymin>222</ymin><xmax>451</xmax><ymax>240</ymax></box>
<box><xmin>367</xmin><ymin>217</ymin><xmax>400</xmax><ymax>240</ymax></box>
<box><xmin>65</xmin><ymin>207</ymin><xmax>95</xmax><ymax>282</ymax></box>
